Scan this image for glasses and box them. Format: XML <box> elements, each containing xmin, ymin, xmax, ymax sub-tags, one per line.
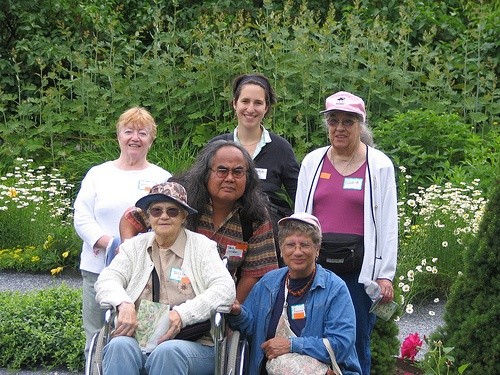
<box><xmin>327</xmin><ymin>117</ymin><xmax>359</xmax><ymax>127</ymax></box>
<box><xmin>209</xmin><ymin>167</ymin><xmax>249</xmax><ymax>178</ymax></box>
<box><xmin>147</xmin><ymin>208</ymin><xmax>183</xmax><ymax>217</ymax></box>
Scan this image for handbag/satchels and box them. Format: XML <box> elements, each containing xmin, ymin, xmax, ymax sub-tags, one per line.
<box><xmin>169</xmin><ymin>319</ymin><xmax>211</xmax><ymax>343</ymax></box>
<box><xmin>265</xmin><ymin>314</ymin><xmax>328</xmax><ymax>375</ymax></box>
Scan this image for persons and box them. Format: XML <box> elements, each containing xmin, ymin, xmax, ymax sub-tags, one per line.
<box><xmin>205</xmin><ymin>74</ymin><xmax>300</xmax><ymax>268</ymax></box>
<box><xmin>115</xmin><ymin>139</ymin><xmax>279</xmax><ymax>339</ymax></box>
<box><xmin>295</xmin><ymin>91</ymin><xmax>398</xmax><ymax>375</ymax></box>
<box><xmin>227</xmin><ymin>212</ymin><xmax>363</xmax><ymax>375</ymax></box>
<box><xmin>93</xmin><ymin>182</ymin><xmax>236</xmax><ymax>375</ymax></box>
<box><xmin>74</xmin><ymin>107</ymin><xmax>174</xmax><ymax>373</ymax></box>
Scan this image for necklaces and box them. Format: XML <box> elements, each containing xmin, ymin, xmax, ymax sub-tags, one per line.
<box><xmin>286</xmin><ymin>269</ymin><xmax>316</xmax><ymax>296</ymax></box>
<box><xmin>333</xmin><ymin>154</ymin><xmax>355</xmax><ymax>175</ymax></box>
<box><xmin>241</xmin><ymin>140</ymin><xmax>260</xmax><ymax>147</ymax></box>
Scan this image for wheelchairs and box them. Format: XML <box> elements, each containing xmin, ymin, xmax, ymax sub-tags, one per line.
<box><xmin>84</xmin><ymin>303</ymin><xmax>253</xmax><ymax>375</ymax></box>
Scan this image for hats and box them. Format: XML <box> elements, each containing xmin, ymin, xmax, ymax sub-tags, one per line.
<box><xmin>135</xmin><ymin>181</ymin><xmax>198</xmax><ymax>216</ymax></box>
<box><xmin>279</xmin><ymin>212</ymin><xmax>322</xmax><ymax>235</ymax></box>
<box><xmin>320</xmin><ymin>91</ymin><xmax>366</xmax><ymax>125</ymax></box>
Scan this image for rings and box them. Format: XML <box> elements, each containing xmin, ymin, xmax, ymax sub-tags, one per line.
<box><xmin>385</xmin><ymin>291</ymin><xmax>389</xmax><ymax>294</ymax></box>
<box><xmin>388</xmin><ymin>292</ymin><xmax>392</xmax><ymax>295</ymax></box>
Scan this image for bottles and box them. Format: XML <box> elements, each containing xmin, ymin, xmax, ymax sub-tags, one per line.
<box><xmin>363</xmin><ymin>277</ymin><xmax>383</xmax><ymax>303</ymax></box>
